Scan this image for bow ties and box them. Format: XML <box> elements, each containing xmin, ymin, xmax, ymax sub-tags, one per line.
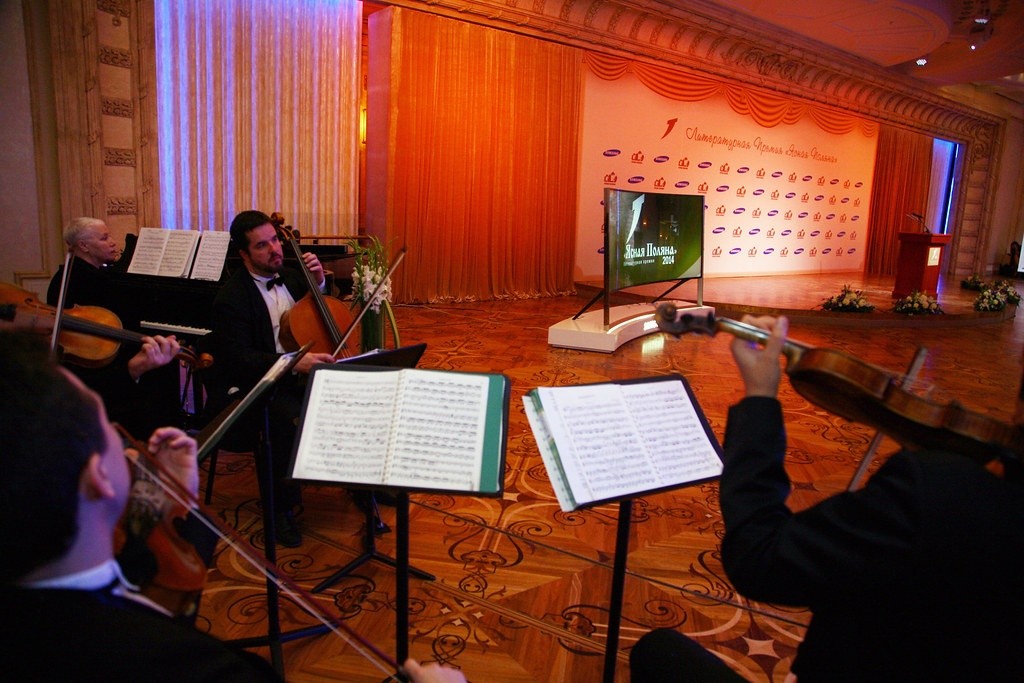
<box><xmin>264</xmin><ymin>277</ymin><xmax>285</xmax><ymax>289</ymax></box>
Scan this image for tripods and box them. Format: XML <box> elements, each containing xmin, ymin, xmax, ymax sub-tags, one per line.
<box><xmin>291</xmin><ymin>344</ymin><xmax>436</xmax><ymax>594</ymax></box>
<box><xmin>196</xmin><ymin>337</ymin><xmax>341</xmax><ymax>683</ymax></box>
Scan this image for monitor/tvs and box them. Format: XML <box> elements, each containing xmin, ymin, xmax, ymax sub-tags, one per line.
<box><xmin>603</xmin><ymin>187</ymin><xmax>705</xmax><ymax>293</ymax></box>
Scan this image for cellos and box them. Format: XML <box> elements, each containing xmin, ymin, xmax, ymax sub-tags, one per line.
<box><xmin>272</xmin><ymin>211</ymin><xmax>363</xmax><ymax>368</ymax></box>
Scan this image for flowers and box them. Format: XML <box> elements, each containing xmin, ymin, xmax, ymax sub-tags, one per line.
<box><xmin>972</xmin><ymin>289</ymin><xmax>1008</xmax><ymax>311</ymax></box>
<box><xmin>892</xmin><ymin>288</ymin><xmax>945</xmax><ymax>315</ymax></box>
<box><xmin>810</xmin><ymin>284</ymin><xmax>877</xmax><ymax>314</ymax></box>
<box><xmin>347</xmin><ymin>232</ymin><xmax>391</xmax><ymax>355</ymax></box>
<box><xmin>960</xmin><ymin>272</ymin><xmax>1021</xmax><ymax>303</ymax></box>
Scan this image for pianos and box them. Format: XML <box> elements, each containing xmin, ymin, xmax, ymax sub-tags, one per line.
<box><xmin>113</xmin><ymin>232</ymin><xmax>385</xmax><ymax>453</ymax></box>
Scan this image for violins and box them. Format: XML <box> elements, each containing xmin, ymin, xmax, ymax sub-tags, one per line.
<box><xmin>0</xmin><ymin>281</ymin><xmax>212</xmax><ymax>363</ymax></box>
<box><xmin>656</xmin><ymin>303</ymin><xmax>1023</xmax><ymax>465</ymax></box>
<box><xmin>106</xmin><ymin>439</ymin><xmax>209</xmax><ymax>616</ymax></box>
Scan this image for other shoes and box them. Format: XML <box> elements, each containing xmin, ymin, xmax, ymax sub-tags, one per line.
<box><xmin>268</xmin><ymin>509</ymin><xmax>304</xmax><ymax>548</ymax></box>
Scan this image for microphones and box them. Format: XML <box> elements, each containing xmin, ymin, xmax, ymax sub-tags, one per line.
<box><xmin>911</xmin><ymin>213</ymin><xmax>930</xmax><ymax>233</ymax></box>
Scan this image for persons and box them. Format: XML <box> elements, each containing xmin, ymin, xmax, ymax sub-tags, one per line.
<box><xmin>631</xmin><ymin>316</ymin><xmax>1024</xmax><ymax>683</ymax></box>
<box><xmin>214</xmin><ymin>210</ymin><xmax>337</xmax><ymax>547</ymax></box>
<box><xmin>47</xmin><ymin>216</ymin><xmax>174</xmax><ymax>427</ymax></box>
<box><xmin>0</xmin><ymin>331</ymin><xmax>181</xmax><ymax>437</ymax></box>
<box><xmin>0</xmin><ymin>350</ymin><xmax>466</xmax><ymax>683</ymax></box>
